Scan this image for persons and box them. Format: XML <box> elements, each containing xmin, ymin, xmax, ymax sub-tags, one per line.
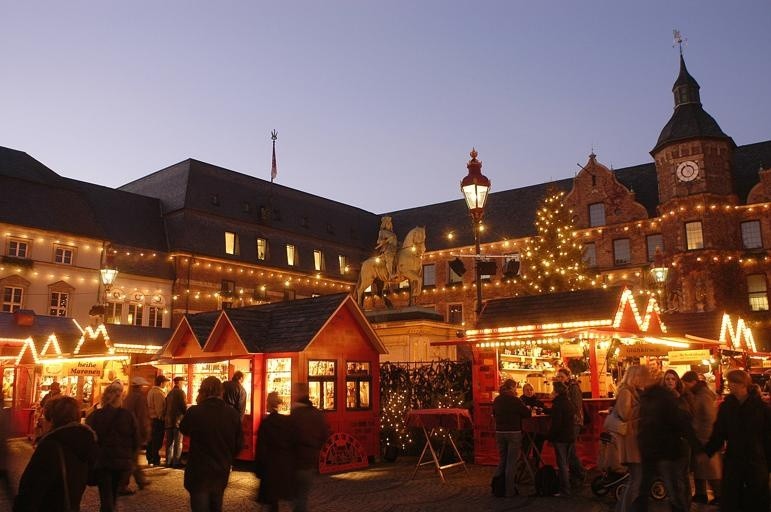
<box><xmin>377</xmin><ymin>215</ymin><xmax>399</xmax><ymax>280</ymax></box>
<box><xmin>10</xmin><ymin>395</ymin><xmax>95</xmax><ymax>512</ymax></box>
<box><xmin>117</xmin><ymin>377</ymin><xmax>153</xmax><ymax>497</ymax></box>
<box><xmin>166</xmin><ymin>376</ymin><xmax>187</xmax><ymax>468</ymax></box>
<box><xmin>40</xmin><ymin>382</ymin><xmax>61</xmax><ymax>408</ymax></box>
<box><xmin>642</xmin><ymin>357</ymin><xmax>665</xmax><ymax>389</ymax></box>
<box><xmin>516</xmin><ymin>383</ymin><xmax>547</xmax><ymax>470</ymax></box>
<box><xmin>223</xmin><ymin>370</ymin><xmax>247</xmax><ymax>423</ymax></box>
<box><xmin>251</xmin><ymin>392</ymin><xmax>294</xmax><ymax>512</ymax></box>
<box><xmin>179</xmin><ymin>376</ymin><xmax>242</xmax><ymax>512</ymax></box>
<box><xmin>674</xmin><ymin>364</ymin><xmax>724</xmax><ymax>508</ymax></box>
<box><xmin>84</xmin><ymin>384</ymin><xmax>139</xmax><ymax>512</ymax></box>
<box><xmin>145</xmin><ymin>375</ymin><xmax>170</xmax><ymax>468</ymax></box>
<box><xmin>555</xmin><ymin>367</ymin><xmax>588</xmax><ymax>484</ymax></box>
<box><xmin>608</xmin><ymin>361</ymin><xmax>650</xmax><ymax>511</ymax></box>
<box><xmin>288</xmin><ymin>383</ymin><xmax>330</xmax><ymax>512</ymax></box>
<box><xmin>700</xmin><ymin>369</ymin><xmax>770</xmax><ymax>512</ymax></box>
<box><xmin>492</xmin><ymin>379</ymin><xmax>531</xmax><ymax>498</ymax></box>
<box><xmin>658</xmin><ymin>367</ymin><xmax>704</xmax><ymax>507</ymax></box>
<box><xmin>537</xmin><ymin>378</ymin><xmax>580</xmax><ymax>492</ymax></box>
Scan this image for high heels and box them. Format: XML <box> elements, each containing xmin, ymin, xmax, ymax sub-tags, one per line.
<box><xmin>490</xmin><ymin>481</ymin><xmax>496</xmax><ymax>491</ymax></box>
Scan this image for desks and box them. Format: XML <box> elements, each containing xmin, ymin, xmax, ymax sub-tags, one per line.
<box><xmin>515</xmin><ymin>414</ymin><xmax>554</xmax><ymax>498</ymax></box>
<box><xmin>403</xmin><ymin>409</ymin><xmax>474</xmax><ymax>485</ymax></box>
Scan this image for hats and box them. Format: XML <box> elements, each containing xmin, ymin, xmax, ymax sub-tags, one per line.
<box><xmin>131</xmin><ymin>376</ymin><xmax>151</xmax><ymax>386</ymax></box>
<box><xmin>295</xmin><ymin>395</ymin><xmax>315</xmax><ymax>408</ymax></box>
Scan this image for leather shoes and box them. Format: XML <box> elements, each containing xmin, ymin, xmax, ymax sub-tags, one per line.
<box><xmin>138</xmin><ymin>482</ymin><xmax>150</xmax><ymax>490</ymax></box>
<box><xmin>118</xmin><ymin>491</ymin><xmax>136</xmax><ymax>497</ymax></box>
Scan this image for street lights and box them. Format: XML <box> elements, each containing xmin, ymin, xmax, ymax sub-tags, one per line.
<box><xmin>461</xmin><ymin>147</ymin><xmax>491</xmax><ymax>315</ymax></box>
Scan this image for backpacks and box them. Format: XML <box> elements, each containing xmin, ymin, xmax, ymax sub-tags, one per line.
<box><xmin>601</xmin><ymin>407</ymin><xmax>634</xmax><ymax>440</ymax></box>
<box><xmin>548</xmin><ymin>408</ymin><xmax>576</xmax><ymax>448</ymax></box>
<box><xmin>692</xmin><ymin>432</ymin><xmax>725</xmax><ymax>461</ymax></box>
